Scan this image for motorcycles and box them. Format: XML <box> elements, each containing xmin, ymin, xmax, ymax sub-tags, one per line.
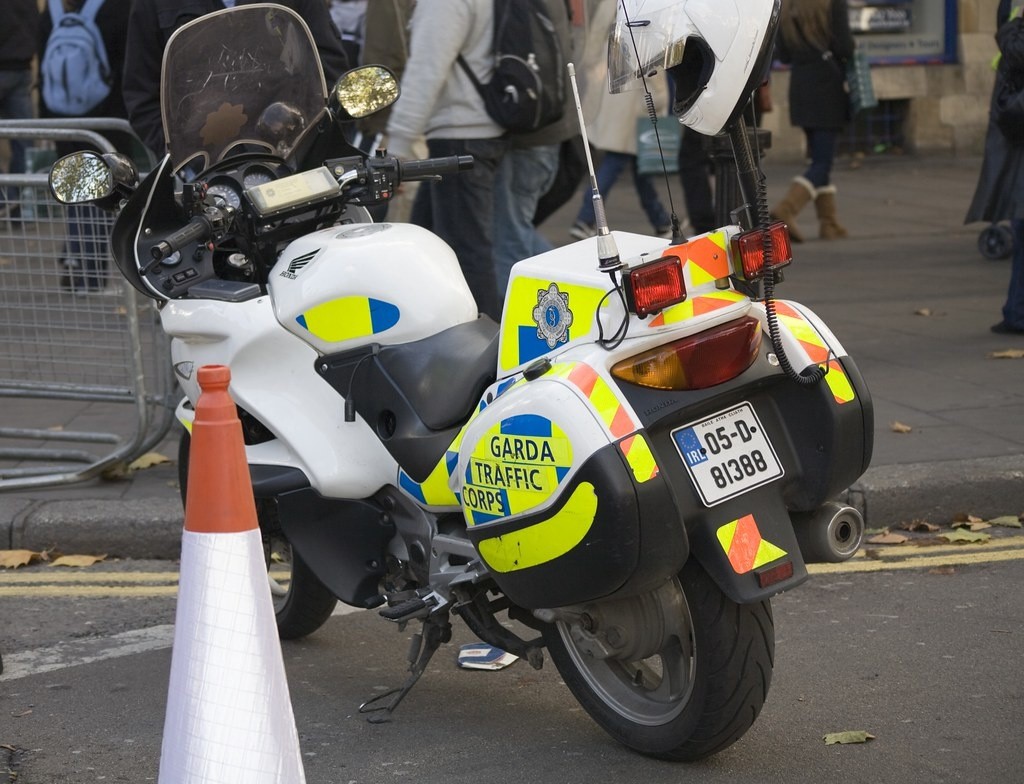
<box><xmin>48</xmin><ymin>3</ymin><xmax>875</xmax><ymax>763</ymax></box>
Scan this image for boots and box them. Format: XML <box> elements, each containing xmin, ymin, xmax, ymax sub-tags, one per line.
<box><xmin>768</xmin><ymin>175</ymin><xmax>819</xmax><ymax>245</ymax></box>
<box><xmin>814</xmin><ymin>183</ymin><xmax>854</xmax><ymax>242</ymax></box>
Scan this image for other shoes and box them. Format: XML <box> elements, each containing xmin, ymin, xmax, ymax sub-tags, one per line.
<box><xmin>59</xmin><ymin>257</ymin><xmax>84</xmax><ymax>292</ymax></box>
<box><xmin>991</xmin><ymin>319</ymin><xmax>1024</xmax><ymax>336</ymax></box>
<box><xmin>568</xmin><ymin>217</ymin><xmax>598</xmax><ymax>241</ymax></box>
<box><xmin>656</xmin><ymin>216</ymin><xmax>690</xmax><ymax>239</ymax></box>
<box><xmin>76</xmin><ymin>287</ymin><xmax>124</xmax><ymax>296</ymax></box>
<box><xmin>0</xmin><ymin>197</ymin><xmax>20</xmax><ymax>218</ymax></box>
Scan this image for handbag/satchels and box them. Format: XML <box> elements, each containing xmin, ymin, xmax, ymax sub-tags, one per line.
<box><xmin>846</xmin><ymin>35</ymin><xmax>879</xmax><ymax>119</ymax></box>
<box><xmin>20</xmin><ymin>138</ymin><xmax>71</xmax><ymax>223</ymax></box>
<box><xmin>991</xmin><ymin>54</ymin><xmax>1024</xmax><ymax>146</ymax></box>
<box><xmin>635</xmin><ymin>116</ymin><xmax>684</xmax><ymax>173</ymax></box>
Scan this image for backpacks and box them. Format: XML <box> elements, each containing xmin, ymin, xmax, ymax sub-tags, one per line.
<box><xmin>456</xmin><ymin>0</ymin><xmax>568</xmax><ymax>135</ymax></box>
<box><xmin>40</xmin><ymin>0</ymin><xmax>117</xmax><ymax>115</ymax></box>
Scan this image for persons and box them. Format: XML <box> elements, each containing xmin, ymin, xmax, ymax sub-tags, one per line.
<box><xmin>569</xmin><ymin>0</ymin><xmax>690</xmax><ymax>242</ymax></box>
<box><xmin>119</xmin><ymin>0</ymin><xmax>235</xmax><ymax>164</ymax></box>
<box><xmin>677</xmin><ymin>70</ymin><xmax>773</xmax><ymax>235</ymax></box>
<box><xmin>769</xmin><ymin>0</ymin><xmax>856</xmax><ymax>244</ymax></box>
<box><xmin>960</xmin><ymin>0</ymin><xmax>1024</xmax><ymax>335</ymax></box>
<box><xmin>37</xmin><ymin>0</ymin><xmax>131</xmax><ymax>296</ymax></box>
<box><xmin>235</xmin><ymin>0</ymin><xmax>369</xmax><ymax>175</ymax></box>
<box><xmin>0</xmin><ymin>0</ymin><xmax>47</xmax><ymax>233</ymax></box>
<box><xmin>376</xmin><ymin>0</ymin><xmax>616</xmax><ymax>324</ymax></box>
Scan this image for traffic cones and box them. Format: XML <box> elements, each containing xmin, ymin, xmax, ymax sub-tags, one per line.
<box><xmin>158</xmin><ymin>365</ymin><xmax>310</xmax><ymax>783</ymax></box>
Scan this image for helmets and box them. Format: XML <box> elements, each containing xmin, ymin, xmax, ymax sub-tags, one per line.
<box><xmin>607</xmin><ymin>0</ymin><xmax>781</xmax><ymax>138</ymax></box>
